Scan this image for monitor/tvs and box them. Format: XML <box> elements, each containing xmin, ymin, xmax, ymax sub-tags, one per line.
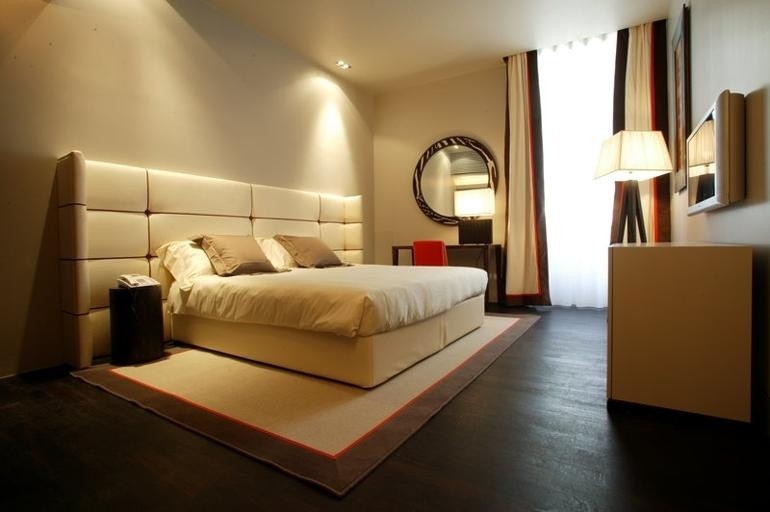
<box><xmin>684</xmin><ymin>89</ymin><xmax>746</xmax><ymax>216</ymax></box>
<box><xmin>458</xmin><ymin>219</ymin><xmax>493</xmax><ymax>245</ymax></box>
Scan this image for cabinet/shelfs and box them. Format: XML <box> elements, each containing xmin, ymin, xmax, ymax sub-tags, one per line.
<box><xmin>445</xmin><ymin>245</ymin><xmax>504</xmax><ymax>312</ymax></box>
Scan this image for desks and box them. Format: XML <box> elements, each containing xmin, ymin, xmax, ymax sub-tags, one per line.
<box><xmin>604</xmin><ymin>242</ymin><xmax>751</xmax><ymax>424</ymax></box>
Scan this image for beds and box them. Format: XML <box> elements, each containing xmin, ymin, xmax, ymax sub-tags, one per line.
<box><xmin>55</xmin><ymin>151</ymin><xmax>491</xmax><ymax>389</ymax></box>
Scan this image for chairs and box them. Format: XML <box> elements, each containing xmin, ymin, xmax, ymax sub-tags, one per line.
<box><xmin>412</xmin><ymin>241</ymin><xmax>449</xmax><ymax>267</ymax></box>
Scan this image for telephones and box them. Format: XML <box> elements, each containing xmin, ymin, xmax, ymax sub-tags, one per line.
<box><xmin>116</xmin><ymin>273</ymin><xmax>161</xmax><ymax>288</ymax></box>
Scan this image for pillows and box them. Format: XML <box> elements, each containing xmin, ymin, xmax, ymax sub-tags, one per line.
<box><xmin>274</xmin><ymin>233</ymin><xmax>344</xmax><ymax>268</ymax></box>
<box><xmin>188</xmin><ymin>231</ymin><xmax>278</xmax><ymax>276</ymax></box>
<box><xmin>155</xmin><ymin>239</ymin><xmax>215</xmax><ymax>293</ymax></box>
<box><xmin>258</xmin><ymin>233</ymin><xmax>301</xmax><ymax>271</ymax></box>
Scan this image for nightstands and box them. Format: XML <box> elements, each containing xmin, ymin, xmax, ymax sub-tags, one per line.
<box><xmin>109</xmin><ymin>285</ymin><xmax>160</xmax><ymax>365</ymax></box>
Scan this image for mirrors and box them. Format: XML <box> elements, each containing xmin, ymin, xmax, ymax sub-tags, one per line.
<box><xmin>411</xmin><ymin>135</ymin><xmax>498</xmax><ymax>226</ymax></box>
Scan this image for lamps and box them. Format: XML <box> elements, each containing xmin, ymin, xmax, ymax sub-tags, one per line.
<box><xmin>453</xmin><ymin>188</ymin><xmax>497</xmax><ymax>244</ymax></box>
<box><xmin>687</xmin><ymin>119</ymin><xmax>716</xmax><ymax>203</ymax></box>
<box><xmin>593</xmin><ymin>130</ymin><xmax>674</xmax><ymax>244</ymax></box>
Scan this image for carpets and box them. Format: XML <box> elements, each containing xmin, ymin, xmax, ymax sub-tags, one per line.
<box><xmin>70</xmin><ymin>312</ymin><xmax>541</xmax><ymax>497</ymax></box>
